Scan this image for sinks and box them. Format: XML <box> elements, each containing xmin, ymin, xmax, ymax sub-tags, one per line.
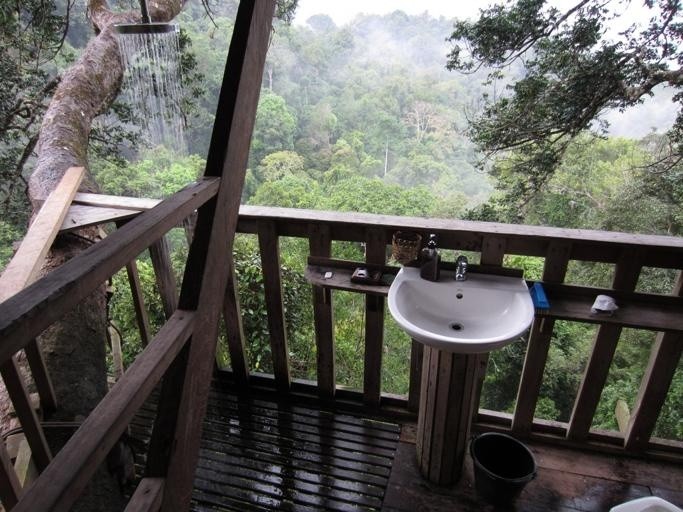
<box><xmin>387</xmin><ymin>267</ymin><xmax>535</xmax><ymax>354</ymax></box>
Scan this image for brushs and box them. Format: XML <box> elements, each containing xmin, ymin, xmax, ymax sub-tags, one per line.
<box><xmin>529</xmin><ymin>282</ymin><xmax>551</xmax><ymax>316</ymax></box>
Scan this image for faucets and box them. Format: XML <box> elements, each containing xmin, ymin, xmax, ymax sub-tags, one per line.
<box><xmin>455</xmin><ymin>256</ymin><xmax>468</xmax><ymax>281</ymax></box>
